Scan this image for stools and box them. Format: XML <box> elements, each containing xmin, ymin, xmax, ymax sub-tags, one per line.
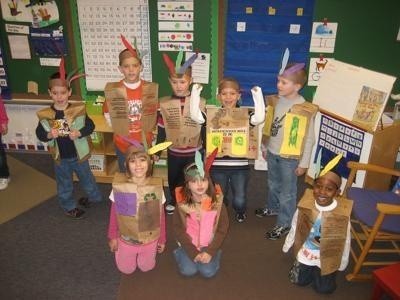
<box><xmin>372</xmin><ymin>264</ymin><xmax>400</xmax><ymax>299</ymax></box>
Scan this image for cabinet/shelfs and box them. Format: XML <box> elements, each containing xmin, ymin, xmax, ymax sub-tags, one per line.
<box><xmin>303</xmin><ymin>104</ymin><xmax>399</xmax><ymax>196</ymax></box>
<box><xmin>69</xmin><ymin>113</ymin><xmax>170</xmax><ymax>186</ymax></box>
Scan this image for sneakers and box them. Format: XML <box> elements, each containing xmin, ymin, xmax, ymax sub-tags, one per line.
<box><xmin>264</xmin><ymin>223</ymin><xmax>291</xmax><ymax>241</ymax></box>
<box><xmin>165</xmin><ymin>203</ymin><xmax>176</xmax><ymax>215</ymax></box>
<box><xmin>254</xmin><ymin>207</ymin><xmax>280</xmax><ymax>218</ymax></box>
<box><xmin>78</xmin><ymin>197</ymin><xmax>102</xmax><ymax>207</ymax></box>
<box><xmin>235</xmin><ymin>211</ymin><xmax>248</xmax><ymax>223</ymax></box>
<box><xmin>0</xmin><ymin>176</ymin><xmax>11</xmax><ymax>190</ymax></box>
<box><xmin>63</xmin><ymin>208</ymin><xmax>87</xmax><ymax>219</ymax></box>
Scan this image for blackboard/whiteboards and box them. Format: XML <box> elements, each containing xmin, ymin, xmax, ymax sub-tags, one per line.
<box><xmin>0</xmin><ymin>0</ymin><xmax>400</xmax><ymax>113</ymax></box>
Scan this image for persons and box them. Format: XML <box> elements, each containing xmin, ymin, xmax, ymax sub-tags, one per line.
<box><xmin>172</xmin><ymin>162</ymin><xmax>230</xmax><ymax>278</ymax></box>
<box><xmin>152</xmin><ymin>62</ymin><xmax>206</xmax><ymax>214</ymax></box>
<box><xmin>103</xmin><ymin>49</ymin><xmax>161</xmax><ymax>172</ymax></box>
<box><xmin>0</xmin><ymin>86</ymin><xmax>12</xmax><ymax>190</ymax></box>
<box><xmin>282</xmin><ymin>170</ymin><xmax>351</xmax><ymax>294</ymax></box>
<box><xmin>256</xmin><ymin>65</ymin><xmax>317</xmax><ymax>240</ymax></box>
<box><xmin>190</xmin><ymin>78</ymin><xmax>264</xmax><ymax>222</ymax></box>
<box><xmin>36</xmin><ymin>71</ymin><xmax>104</xmax><ymax>219</ymax></box>
<box><xmin>108</xmin><ymin>143</ymin><xmax>167</xmax><ymax>274</ymax></box>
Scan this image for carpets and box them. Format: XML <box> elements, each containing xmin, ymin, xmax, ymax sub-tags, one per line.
<box><xmin>0</xmin><ymin>151</ymin><xmax>59</xmax><ymax>228</ymax></box>
<box><xmin>116</xmin><ymin>169</ymin><xmax>373</xmax><ymax>299</ymax></box>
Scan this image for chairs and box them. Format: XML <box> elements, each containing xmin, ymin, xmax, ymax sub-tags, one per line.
<box><xmin>337</xmin><ymin>159</ymin><xmax>400</xmax><ymax>282</ymax></box>
